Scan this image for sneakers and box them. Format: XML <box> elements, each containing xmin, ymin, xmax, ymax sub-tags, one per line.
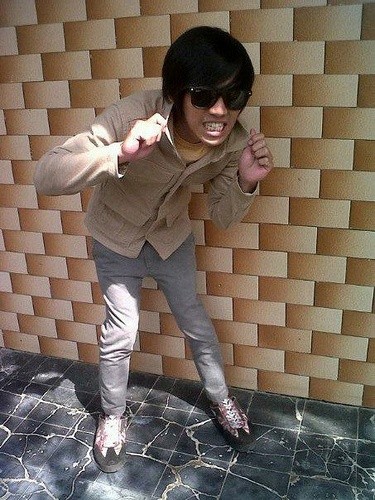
<box><xmin>210</xmin><ymin>395</ymin><xmax>256</xmax><ymax>454</ymax></box>
<box><xmin>93</xmin><ymin>413</ymin><xmax>127</xmax><ymax>472</ymax></box>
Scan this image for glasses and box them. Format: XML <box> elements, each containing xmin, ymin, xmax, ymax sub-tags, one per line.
<box><xmin>184</xmin><ymin>87</ymin><xmax>252</xmax><ymax>112</ymax></box>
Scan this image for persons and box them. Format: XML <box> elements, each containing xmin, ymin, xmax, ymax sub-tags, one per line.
<box><xmin>32</xmin><ymin>26</ymin><xmax>275</xmax><ymax>473</ymax></box>
